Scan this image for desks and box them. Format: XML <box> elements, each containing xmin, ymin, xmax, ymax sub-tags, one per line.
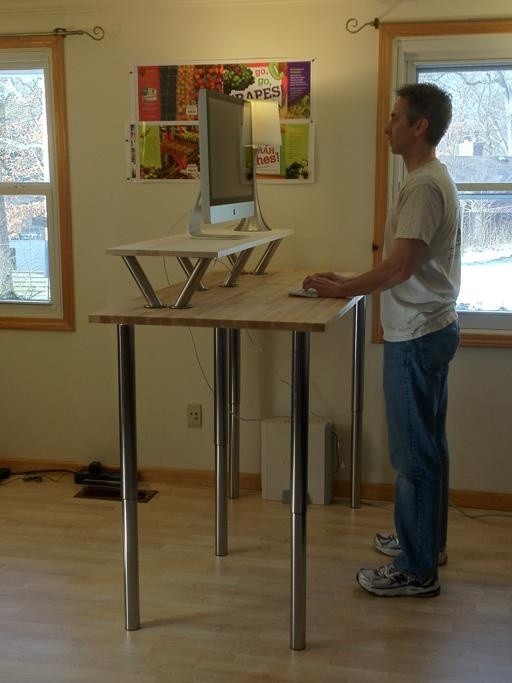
<box><xmin>87</xmin><ymin>226</ymin><xmax>366</xmax><ymax>654</ymax></box>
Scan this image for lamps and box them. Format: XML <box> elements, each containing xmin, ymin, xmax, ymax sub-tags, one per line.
<box><xmin>233</xmin><ymin>99</ymin><xmax>283</xmax><ymax>232</ymax></box>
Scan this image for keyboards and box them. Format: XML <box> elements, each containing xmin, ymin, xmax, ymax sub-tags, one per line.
<box><xmin>288</xmin><ymin>284</ymin><xmax>318</xmax><ymax>298</ymax></box>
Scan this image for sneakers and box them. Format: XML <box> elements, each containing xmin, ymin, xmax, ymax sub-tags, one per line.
<box><xmin>356</xmin><ymin>563</ymin><xmax>441</xmax><ymax>598</ymax></box>
<box><xmin>371</xmin><ymin>530</ymin><xmax>449</xmax><ymax>565</ymax></box>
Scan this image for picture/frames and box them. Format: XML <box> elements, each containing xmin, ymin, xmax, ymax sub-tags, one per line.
<box><xmin>122</xmin><ymin>57</ymin><xmax>317</xmax><ymax>185</ymax></box>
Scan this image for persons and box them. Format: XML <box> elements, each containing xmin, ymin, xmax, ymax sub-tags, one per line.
<box><xmin>300</xmin><ymin>81</ymin><xmax>468</xmax><ymax>599</ymax></box>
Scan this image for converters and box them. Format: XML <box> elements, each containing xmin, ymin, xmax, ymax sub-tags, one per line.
<box><xmin>0</xmin><ymin>468</ymin><xmax>12</xmax><ymax>481</ymax></box>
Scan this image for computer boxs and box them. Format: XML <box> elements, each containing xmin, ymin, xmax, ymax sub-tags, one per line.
<box><xmin>260</xmin><ymin>419</ymin><xmax>334</xmax><ymax>506</ymax></box>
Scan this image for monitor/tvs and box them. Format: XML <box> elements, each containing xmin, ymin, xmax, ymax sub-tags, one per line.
<box><xmin>189</xmin><ymin>89</ymin><xmax>257</xmax><ymax>240</ymax></box>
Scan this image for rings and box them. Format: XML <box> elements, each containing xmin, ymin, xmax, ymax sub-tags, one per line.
<box><xmin>310</xmin><ymin>277</ymin><xmax>317</xmax><ymax>283</ymax></box>
